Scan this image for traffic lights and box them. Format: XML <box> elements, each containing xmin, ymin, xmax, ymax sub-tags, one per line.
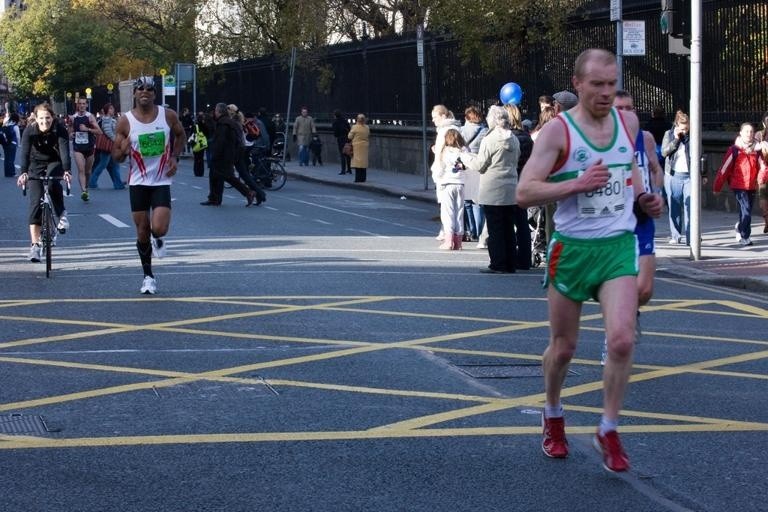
<box><xmin>660</xmin><ymin>0</ymin><xmax>681</xmax><ymax>36</ymax></box>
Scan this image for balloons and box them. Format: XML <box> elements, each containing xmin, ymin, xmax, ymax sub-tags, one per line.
<box><xmin>500</xmin><ymin>82</ymin><xmax>523</xmax><ymax>105</ymax></box>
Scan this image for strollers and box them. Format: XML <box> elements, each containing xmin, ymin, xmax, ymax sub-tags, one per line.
<box><xmin>519</xmin><ymin>204</ymin><xmax>547</xmax><ymax>267</ymax></box>
<box><xmin>271</xmin><ymin>131</ymin><xmax>290</xmax><ymax>163</ymax></box>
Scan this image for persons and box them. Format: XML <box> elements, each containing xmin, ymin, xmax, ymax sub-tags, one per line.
<box><xmin>712</xmin><ymin>122</ymin><xmax>768</xmax><ymax>246</ymax></box>
<box><xmin>601</xmin><ymin>90</ymin><xmax>664</xmax><ymax>366</ymax></box>
<box><xmin>112</xmin><ymin>77</ymin><xmax>185</xmax><ymax>293</ymax></box>
<box><xmin>1</xmin><ymin>96</ymin><xmax>128</xmax><ymax>200</ymax></box>
<box><xmin>516</xmin><ymin>48</ymin><xmax>664</xmax><ymax>471</ymax></box>
<box><xmin>428</xmin><ymin>91</ymin><xmax>578</xmax><ymax>274</ymax></box>
<box><xmin>347</xmin><ymin>114</ymin><xmax>370</xmax><ymax>182</ymax></box>
<box><xmin>660</xmin><ymin>110</ymin><xmax>708</xmax><ymax>246</ymax></box>
<box><xmin>164</xmin><ymin>102</ymin><xmax>321</xmax><ymax>207</ymax></box>
<box><xmin>754</xmin><ymin>111</ymin><xmax>768</xmax><ymax>233</ymax></box>
<box><xmin>18</xmin><ymin>102</ymin><xmax>71</xmax><ymax>263</ymax></box>
<box><xmin>332</xmin><ymin>108</ymin><xmax>352</xmax><ymax>175</ymax></box>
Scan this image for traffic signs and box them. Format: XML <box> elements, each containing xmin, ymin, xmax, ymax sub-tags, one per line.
<box><xmin>610</xmin><ymin>0</ymin><xmax>621</xmax><ymax>22</ymax></box>
<box><xmin>622</xmin><ymin>20</ymin><xmax>646</xmax><ymax>58</ymax></box>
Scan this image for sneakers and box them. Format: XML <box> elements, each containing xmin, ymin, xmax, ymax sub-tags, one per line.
<box><xmin>114</xmin><ymin>181</ymin><xmax>129</xmax><ymax>191</ymax></box>
<box><xmin>541</xmin><ymin>405</ymin><xmax>569</xmax><ymax>459</ymax></box>
<box><xmin>592</xmin><ymin>426</ymin><xmax>631</xmax><ymax>474</ymax></box>
<box><xmin>479</xmin><ymin>267</ymin><xmax>507</xmax><ymax>273</ymax></box>
<box><xmin>57</xmin><ymin>211</ymin><xmax>70</xmax><ymax>234</ymax></box>
<box><xmin>151</xmin><ymin>231</ymin><xmax>166</xmax><ymax>259</ymax></box>
<box><xmin>668</xmin><ymin>237</ymin><xmax>681</xmax><ymax>244</ymax></box>
<box><xmin>246</xmin><ymin>191</ymin><xmax>266</xmax><ymax>206</ymax></box>
<box><xmin>200</xmin><ymin>200</ymin><xmax>222</xmax><ymax>205</ymax></box>
<box><xmin>81</xmin><ymin>190</ymin><xmax>90</xmax><ymax>202</ymax></box>
<box><xmin>29</xmin><ymin>243</ymin><xmax>42</xmax><ymax>263</ymax></box>
<box><xmin>735</xmin><ymin>221</ymin><xmax>753</xmax><ymax>246</ymax></box>
<box><xmin>463</xmin><ymin>235</ymin><xmax>488</xmax><ymax>249</ymax></box>
<box><xmin>140</xmin><ymin>273</ymin><xmax>157</xmax><ymax>294</ymax></box>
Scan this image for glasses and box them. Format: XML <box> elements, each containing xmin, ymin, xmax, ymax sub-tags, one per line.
<box><xmin>136</xmin><ymin>85</ymin><xmax>155</xmax><ymax>91</ymax></box>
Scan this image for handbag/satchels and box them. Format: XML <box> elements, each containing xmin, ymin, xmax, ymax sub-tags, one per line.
<box><xmin>188</xmin><ymin>131</ymin><xmax>209</xmax><ymax>153</ymax></box>
<box><xmin>243</xmin><ymin>119</ymin><xmax>260</xmax><ymax>137</ymax></box>
<box><xmin>95</xmin><ymin>134</ymin><xmax>114</xmax><ymax>153</ymax></box>
<box><xmin>342</xmin><ymin>143</ymin><xmax>353</xmax><ymax>156</ymax></box>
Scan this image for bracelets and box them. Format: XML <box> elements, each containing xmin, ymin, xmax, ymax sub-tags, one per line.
<box><xmin>637</xmin><ymin>192</ymin><xmax>646</xmax><ymax>213</ymax></box>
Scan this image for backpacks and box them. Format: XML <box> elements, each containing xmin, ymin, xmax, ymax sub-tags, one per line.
<box><xmin>0</xmin><ymin>124</ymin><xmax>17</xmax><ymax>146</ymax></box>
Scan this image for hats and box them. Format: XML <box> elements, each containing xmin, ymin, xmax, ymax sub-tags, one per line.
<box><xmin>552</xmin><ymin>91</ymin><xmax>578</xmax><ymax>108</ymax></box>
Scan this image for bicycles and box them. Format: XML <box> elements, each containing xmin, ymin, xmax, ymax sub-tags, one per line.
<box><xmin>224</xmin><ymin>145</ymin><xmax>287</xmax><ymax>191</ymax></box>
<box><xmin>22</xmin><ymin>176</ymin><xmax>70</xmax><ymax>278</ymax></box>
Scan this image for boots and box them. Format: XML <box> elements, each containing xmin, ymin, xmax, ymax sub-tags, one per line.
<box><xmin>339</xmin><ymin>157</ymin><xmax>352</xmax><ymax>175</ymax></box>
<box><xmin>434</xmin><ymin>233</ymin><xmax>464</xmax><ymax>250</ymax></box>
